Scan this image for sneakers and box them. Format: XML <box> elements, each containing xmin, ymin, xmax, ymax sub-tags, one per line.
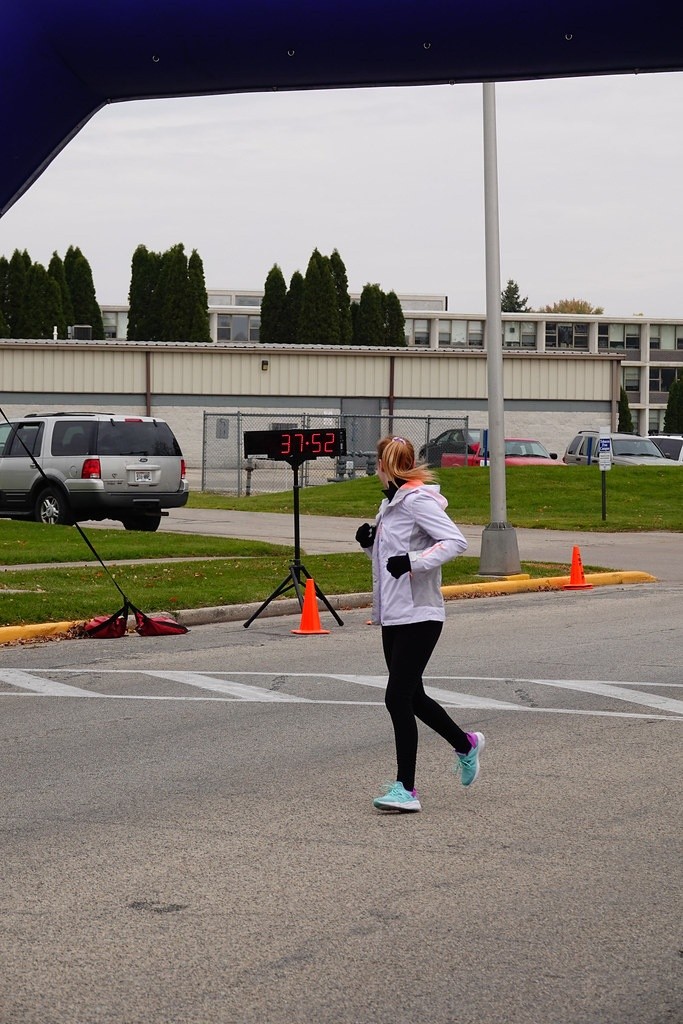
<box><xmin>373</xmin><ymin>781</ymin><xmax>421</xmax><ymax>810</ymax></box>
<box><xmin>454</xmin><ymin>732</ymin><xmax>485</xmax><ymax>786</ymax></box>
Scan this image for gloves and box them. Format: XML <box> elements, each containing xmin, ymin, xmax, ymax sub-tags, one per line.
<box><xmin>387</xmin><ymin>554</ymin><xmax>410</xmax><ymax>579</ymax></box>
<box><xmin>355</xmin><ymin>523</ymin><xmax>375</xmax><ymax>547</ymax></box>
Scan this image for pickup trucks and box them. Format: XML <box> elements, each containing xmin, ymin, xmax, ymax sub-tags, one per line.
<box><xmin>440</xmin><ymin>437</ymin><xmax>568</xmax><ymax>469</ymax></box>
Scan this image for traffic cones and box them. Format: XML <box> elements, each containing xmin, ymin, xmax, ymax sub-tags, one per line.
<box><xmin>290</xmin><ymin>577</ymin><xmax>330</xmax><ymax>635</ymax></box>
<box><xmin>562</xmin><ymin>546</ymin><xmax>594</xmax><ymax>590</ymax></box>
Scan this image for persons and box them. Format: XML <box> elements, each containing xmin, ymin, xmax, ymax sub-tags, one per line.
<box><xmin>354</xmin><ymin>437</ymin><xmax>486</xmax><ymax>811</ymax></box>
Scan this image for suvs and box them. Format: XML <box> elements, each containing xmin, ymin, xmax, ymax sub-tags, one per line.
<box><xmin>562</xmin><ymin>430</ymin><xmax>683</xmax><ymax>467</ymax></box>
<box><xmin>0</xmin><ymin>411</ymin><xmax>190</xmax><ymax>532</ymax></box>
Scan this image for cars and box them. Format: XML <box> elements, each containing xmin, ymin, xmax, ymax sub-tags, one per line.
<box><xmin>416</xmin><ymin>427</ymin><xmax>489</xmax><ymax>468</ymax></box>
